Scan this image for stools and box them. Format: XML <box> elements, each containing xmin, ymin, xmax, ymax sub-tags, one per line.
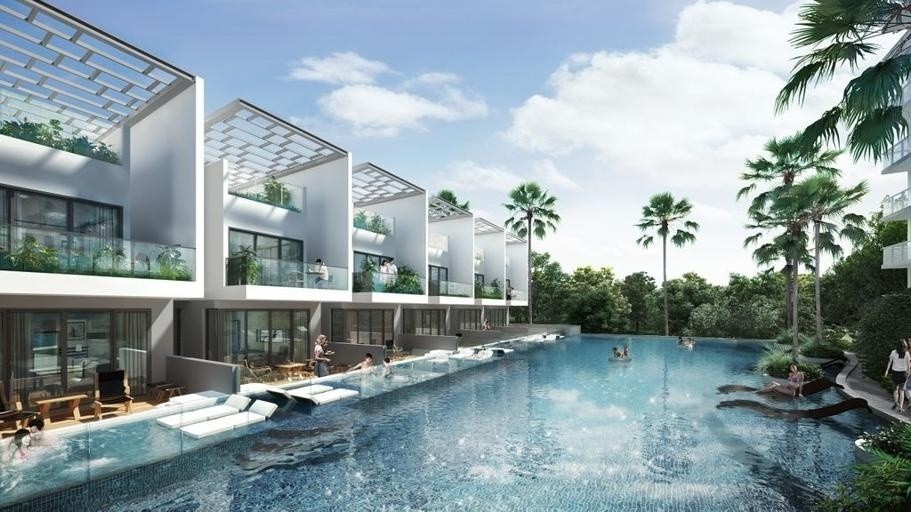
<box><xmin>146</xmin><ymin>382</ymin><xmax>169</xmax><ymax>401</ymax></box>
<box><xmin>156</xmin><ymin>384</ymin><xmax>186</xmax><ymax>404</ymax></box>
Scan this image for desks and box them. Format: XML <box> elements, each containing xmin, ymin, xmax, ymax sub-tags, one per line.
<box><xmin>31</xmin><ymin>391</ymin><xmax>88</xmax><ymax>430</ymax></box>
<box><xmin>273</xmin><ymin>361</ymin><xmax>307</xmax><ymax>382</ymax></box>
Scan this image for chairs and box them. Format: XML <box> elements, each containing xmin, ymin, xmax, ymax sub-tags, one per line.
<box><xmin>307</xmin><ymin>356</ymin><xmax>324</xmax><ymax>375</ymax></box>
<box><xmin>0</xmin><ymin>369</ymin><xmax>40</xmax><ymax>436</ymax></box>
<box><xmin>240</xmin><ymin>356</ymin><xmax>271</xmax><ymax>384</ymax></box>
<box><xmin>89</xmin><ymin>370</ymin><xmax>134</xmax><ymax>421</ymax></box>
